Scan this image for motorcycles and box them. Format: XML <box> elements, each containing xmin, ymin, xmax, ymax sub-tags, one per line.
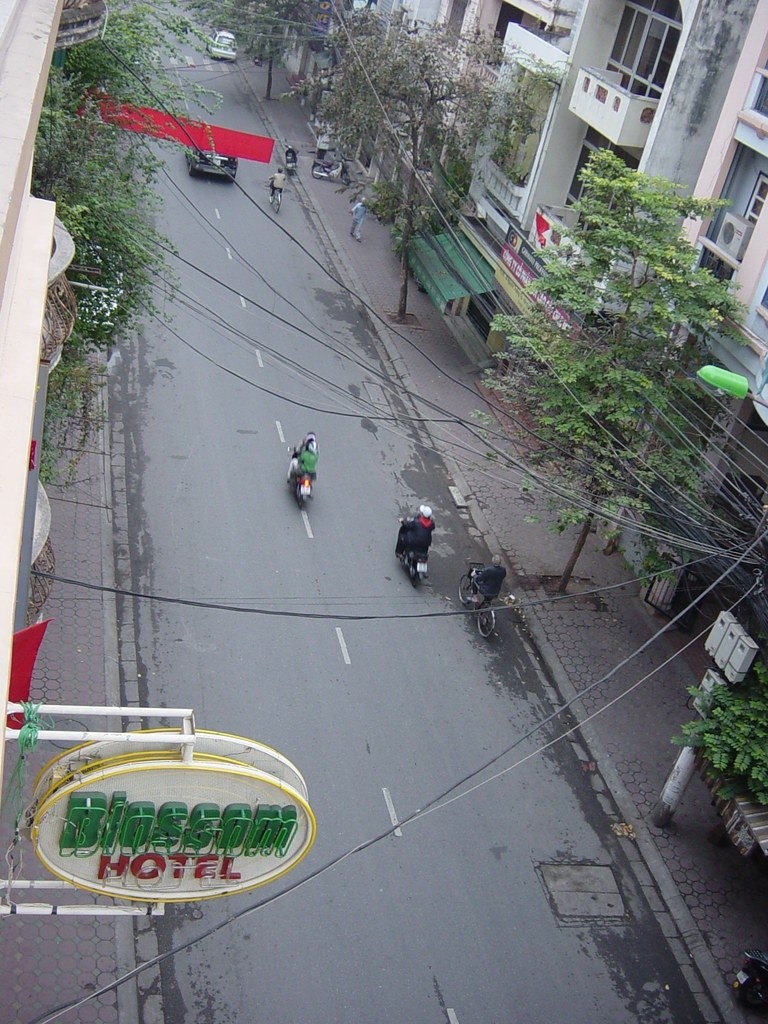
<box><xmin>287</xmin><ymin>432</ymin><xmax>317</xmax><ymax>509</ymax></box>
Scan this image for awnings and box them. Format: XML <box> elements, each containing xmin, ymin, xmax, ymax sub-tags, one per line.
<box><xmin>406</xmin><ymin>230</ymin><xmax>498</xmax><ymax>315</ymax></box>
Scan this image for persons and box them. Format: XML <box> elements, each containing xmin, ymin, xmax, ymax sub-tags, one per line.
<box><xmin>285</xmin><ymin>144</ymin><xmax>298</xmax><ymax>168</ymax></box>
<box><xmin>466</xmin><ymin>554</ymin><xmax>506</xmax><ymax>604</ymax></box>
<box><xmin>348</xmin><ymin>197</ymin><xmax>368</xmax><ymax>243</ymax></box>
<box><xmin>269</xmin><ymin>168</ymin><xmax>285</xmax><ymax>201</ymax></box>
<box><xmin>287</xmin><ymin>439</ymin><xmax>320</xmax><ymax>499</ymax></box>
<box><xmin>316</xmin><ymin>128</ymin><xmax>330</xmax><ymax>160</ymax></box>
<box><xmin>399</xmin><ymin>505</ymin><xmax>435</xmax><ymax>556</ymax></box>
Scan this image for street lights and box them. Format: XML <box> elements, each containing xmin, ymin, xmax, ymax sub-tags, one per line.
<box><xmin>652</xmin><ymin>364</ymin><xmax>768</xmax><ymax>831</ymax></box>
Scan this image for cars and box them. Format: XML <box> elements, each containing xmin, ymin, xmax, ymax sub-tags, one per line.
<box><xmin>184</xmin><ymin>146</ymin><xmax>239</xmax><ymax>183</ymax></box>
<box><xmin>205</xmin><ymin>31</ymin><xmax>237</xmax><ymax>63</ymax></box>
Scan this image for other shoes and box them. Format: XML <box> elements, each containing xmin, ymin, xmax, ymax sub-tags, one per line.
<box><xmin>467</xmin><ymin>597</ymin><xmax>477</xmax><ymax>604</ymax></box>
<box><xmin>356</xmin><ymin>238</ymin><xmax>362</xmax><ymax>243</ymax></box>
<box><xmin>350</xmin><ymin>233</ymin><xmax>354</xmax><ymax>237</ymax></box>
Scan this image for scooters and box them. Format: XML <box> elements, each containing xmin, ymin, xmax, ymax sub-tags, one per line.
<box><xmin>395</xmin><ymin>517</ymin><xmax>429</xmax><ymax>588</ymax></box>
<box><xmin>312</xmin><ymin>154</ymin><xmax>350</xmax><ymax>186</ymax></box>
<box><xmin>282</xmin><ymin>138</ymin><xmax>299</xmax><ymax>176</ymax></box>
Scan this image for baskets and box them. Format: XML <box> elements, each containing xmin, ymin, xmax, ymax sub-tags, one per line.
<box><xmin>468</xmin><ymin>562</ymin><xmax>485</xmax><ymax>578</ymax></box>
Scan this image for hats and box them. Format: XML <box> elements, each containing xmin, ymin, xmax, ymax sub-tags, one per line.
<box><xmin>307</xmin><ymin>439</ymin><xmax>317</xmax><ymax>454</ymax></box>
<box><xmin>420</xmin><ymin>505</ymin><xmax>432</xmax><ymax>519</ymax></box>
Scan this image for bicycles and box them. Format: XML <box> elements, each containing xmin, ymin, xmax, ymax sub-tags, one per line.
<box><xmin>268</xmin><ymin>188</ymin><xmax>282</xmax><ymax>214</ymax></box>
<box><xmin>458</xmin><ymin>561</ymin><xmax>497</xmax><ymax>638</ymax></box>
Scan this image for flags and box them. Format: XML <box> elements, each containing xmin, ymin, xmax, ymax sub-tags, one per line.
<box><xmin>534</xmin><ymin>215</ymin><xmax>549</xmax><ymax>247</ymax></box>
